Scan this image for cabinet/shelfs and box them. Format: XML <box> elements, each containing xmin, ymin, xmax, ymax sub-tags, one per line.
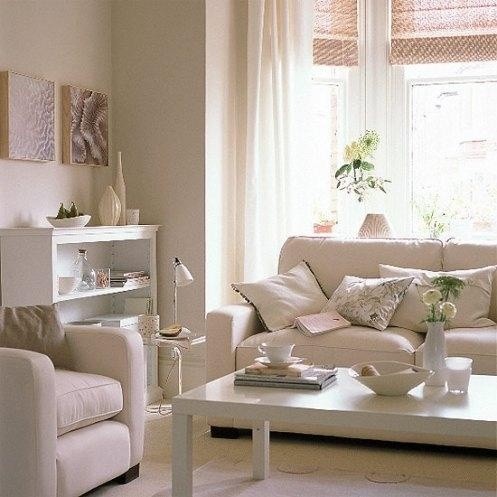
<box><xmin>0</xmin><ymin>225</ymin><xmax>162</xmax><ymax>407</ymax></box>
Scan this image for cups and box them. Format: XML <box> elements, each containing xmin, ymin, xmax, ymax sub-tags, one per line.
<box><xmin>257</xmin><ymin>342</ymin><xmax>295</xmax><ymax>362</ymax></box>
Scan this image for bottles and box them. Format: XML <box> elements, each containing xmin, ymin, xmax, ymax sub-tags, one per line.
<box><xmin>69</xmin><ymin>250</ymin><xmax>96</xmax><ymax>293</ymax></box>
<box><xmin>112</xmin><ymin>151</ymin><xmax>126</xmax><ymax>226</ymax></box>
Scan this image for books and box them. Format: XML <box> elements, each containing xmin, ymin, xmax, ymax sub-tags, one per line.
<box><xmin>106</xmin><ymin>268</ymin><xmax>150</xmax><ymax>288</ymax></box>
<box><xmin>289</xmin><ymin>311</ymin><xmax>351</xmax><ymax>338</ymax></box>
<box><xmin>233</xmin><ymin>362</ymin><xmax>338</xmax><ymax>391</ymax></box>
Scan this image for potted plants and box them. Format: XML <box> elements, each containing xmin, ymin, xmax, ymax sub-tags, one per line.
<box><xmin>312</xmin><ymin>209</ymin><xmax>336</xmax><ymax>234</ymax></box>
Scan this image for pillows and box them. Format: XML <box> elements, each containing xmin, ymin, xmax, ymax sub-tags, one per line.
<box><xmin>232</xmin><ymin>261</ymin><xmax>330</xmax><ymax>332</ymax></box>
<box><xmin>379</xmin><ymin>264</ymin><xmax>497</xmax><ymax>332</ymax></box>
<box><xmin>0</xmin><ymin>304</ymin><xmax>74</xmax><ymax>371</ymax></box>
<box><xmin>320</xmin><ymin>274</ymin><xmax>414</xmax><ymax>331</ymax></box>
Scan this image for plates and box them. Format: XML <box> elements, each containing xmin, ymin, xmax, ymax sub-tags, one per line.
<box><xmin>155</xmin><ymin>332</ymin><xmax>195</xmax><ymax>339</ymax></box>
<box><xmin>46</xmin><ymin>215</ymin><xmax>91</xmax><ymax>228</ymax></box>
<box><xmin>254</xmin><ymin>357</ymin><xmax>303</xmax><ymax>369</ymax></box>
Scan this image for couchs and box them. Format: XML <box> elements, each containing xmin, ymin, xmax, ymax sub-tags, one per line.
<box><xmin>0</xmin><ymin>305</ymin><xmax>145</xmax><ymax>497</ymax></box>
<box><xmin>206</xmin><ymin>235</ymin><xmax>441</xmax><ymax>439</ymax></box>
<box><xmin>415</xmin><ymin>241</ymin><xmax>497</xmax><ymax>450</ymax></box>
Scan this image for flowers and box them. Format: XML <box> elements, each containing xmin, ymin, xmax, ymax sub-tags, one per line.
<box><xmin>336</xmin><ymin>128</ymin><xmax>392</xmax><ymax>214</ymax></box>
<box><xmin>415</xmin><ymin>277</ymin><xmax>466</xmax><ymax>324</ymax></box>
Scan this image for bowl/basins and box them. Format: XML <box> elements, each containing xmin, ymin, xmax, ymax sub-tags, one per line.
<box><xmin>348</xmin><ymin>361</ymin><xmax>436</xmax><ymax>396</ymax></box>
<box><xmin>58</xmin><ymin>277</ymin><xmax>83</xmax><ymax>295</ymax></box>
<box><xmin>444</xmin><ymin>357</ymin><xmax>474</xmax><ymax>371</ymax></box>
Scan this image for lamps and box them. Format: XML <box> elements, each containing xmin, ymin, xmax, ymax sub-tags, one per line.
<box><xmin>156</xmin><ymin>256</ymin><xmax>194</xmax><ymax>340</ymax></box>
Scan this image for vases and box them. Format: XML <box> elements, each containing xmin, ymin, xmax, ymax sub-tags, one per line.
<box><xmin>113</xmin><ymin>151</ymin><xmax>127</xmax><ymax>225</ymax></box>
<box><xmin>357</xmin><ymin>215</ymin><xmax>394</xmax><ymax>238</ymax></box>
<box><xmin>422</xmin><ymin>321</ymin><xmax>447</xmax><ymax>386</ymax></box>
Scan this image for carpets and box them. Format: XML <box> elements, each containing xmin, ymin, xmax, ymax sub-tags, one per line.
<box><xmin>149</xmin><ymin>458</ymin><xmax>497</xmax><ymax>497</ymax></box>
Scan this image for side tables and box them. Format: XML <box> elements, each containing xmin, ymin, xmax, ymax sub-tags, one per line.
<box><xmin>145</xmin><ymin>334</ymin><xmax>207</xmax><ymax>415</ymax></box>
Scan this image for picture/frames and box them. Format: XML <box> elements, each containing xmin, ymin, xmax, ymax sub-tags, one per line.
<box><xmin>2</xmin><ymin>69</ymin><xmax>56</xmax><ymax>163</ymax></box>
<box><xmin>61</xmin><ymin>83</ymin><xmax>109</xmax><ymax>166</ymax></box>
